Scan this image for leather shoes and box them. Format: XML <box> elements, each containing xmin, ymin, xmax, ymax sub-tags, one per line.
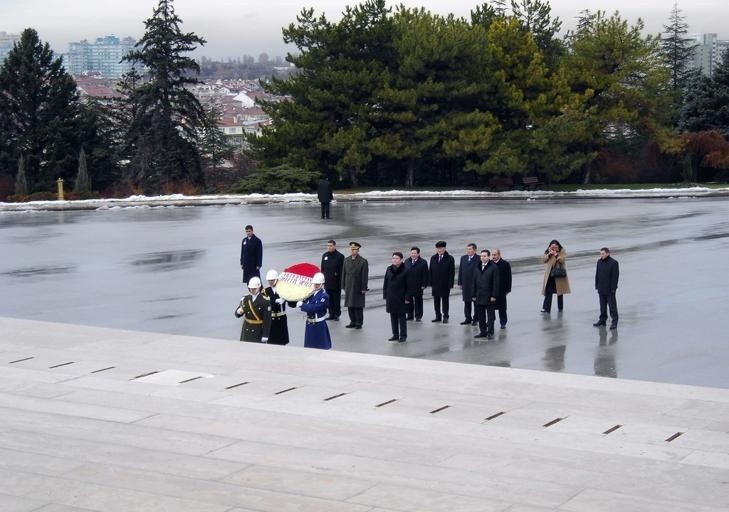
<box><xmin>593</xmin><ymin>321</ymin><xmax>605</xmax><ymax>327</ymax></box>
<box><xmin>609</xmin><ymin>324</ymin><xmax>617</xmax><ymax>330</ymax></box>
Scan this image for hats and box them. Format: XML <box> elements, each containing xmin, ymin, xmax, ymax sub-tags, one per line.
<box><xmin>350</xmin><ymin>242</ymin><xmax>362</xmax><ymax>250</ymax></box>
<box><xmin>435</xmin><ymin>240</ymin><xmax>447</xmax><ymax>248</ymax></box>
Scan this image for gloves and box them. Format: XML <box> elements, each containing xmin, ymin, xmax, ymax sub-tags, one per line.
<box><xmin>276</xmin><ymin>298</ymin><xmax>284</xmax><ymax>305</ymax></box>
<box><xmin>262</xmin><ymin>337</ymin><xmax>269</xmax><ymax>342</ymax></box>
<box><xmin>297</xmin><ymin>301</ymin><xmax>303</xmax><ymax>307</ymax></box>
<box><xmin>237</xmin><ymin>307</ymin><xmax>241</xmax><ymax>315</ymax></box>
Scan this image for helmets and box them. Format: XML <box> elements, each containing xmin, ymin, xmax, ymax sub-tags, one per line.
<box><xmin>247</xmin><ymin>276</ymin><xmax>262</xmax><ymax>289</ymax></box>
<box><xmin>266</xmin><ymin>269</ymin><xmax>279</xmax><ymax>281</ymax></box>
<box><xmin>311</xmin><ymin>273</ymin><xmax>326</xmax><ymax>284</ymax></box>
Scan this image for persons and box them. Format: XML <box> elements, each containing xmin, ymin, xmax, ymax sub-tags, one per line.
<box><xmin>234</xmin><ymin>276</ymin><xmax>271</xmax><ymax>343</ymax></box>
<box><xmin>296</xmin><ymin>272</ymin><xmax>332</xmax><ymax>350</ymax></box>
<box><xmin>265</xmin><ymin>269</ymin><xmax>297</xmax><ymax>345</ymax></box>
<box><xmin>318</xmin><ymin>175</ymin><xmax>334</xmax><ymax>218</ymax></box>
<box><xmin>340</xmin><ymin>242</ymin><xmax>370</xmax><ymax>329</ymax></box>
<box><xmin>321</xmin><ymin>240</ymin><xmax>344</xmax><ymax>321</ymax></box>
<box><xmin>540</xmin><ymin>239</ymin><xmax>571</xmax><ymax>313</ymax></box>
<box><xmin>240</xmin><ymin>225</ymin><xmax>263</xmax><ymax>292</ymax></box>
<box><xmin>383</xmin><ymin>241</ymin><xmax>512</xmax><ymax>343</ymax></box>
<box><xmin>593</xmin><ymin>248</ymin><xmax>619</xmax><ymax>329</ymax></box>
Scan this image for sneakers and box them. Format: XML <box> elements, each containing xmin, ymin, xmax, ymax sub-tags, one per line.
<box><xmin>432</xmin><ymin>316</ymin><xmax>441</xmax><ymax>323</ymax></box>
<box><xmin>541</xmin><ymin>309</ymin><xmax>549</xmax><ymax>314</ymax></box>
<box><xmin>475</xmin><ymin>332</ymin><xmax>485</xmax><ymax>338</ymax></box>
<box><xmin>389</xmin><ymin>335</ymin><xmax>406</xmax><ymax>343</ymax></box>
<box><xmin>488</xmin><ymin>333</ymin><xmax>494</xmax><ymax>339</ymax></box>
<box><xmin>500</xmin><ymin>325</ymin><xmax>506</xmax><ymax>330</ymax></box>
<box><xmin>460</xmin><ymin>319</ymin><xmax>472</xmax><ymax>325</ymax></box>
<box><xmin>405</xmin><ymin>316</ymin><xmax>421</xmax><ymax>322</ymax></box>
<box><xmin>345</xmin><ymin>322</ymin><xmax>362</xmax><ymax>329</ymax></box>
<box><xmin>442</xmin><ymin>317</ymin><xmax>449</xmax><ymax>323</ymax></box>
<box><xmin>472</xmin><ymin>321</ymin><xmax>477</xmax><ymax>326</ymax></box>
<box><xmin>327</xmin><ymin>314</ymin><xmax>339</xmax><ymax>321</ymax></box>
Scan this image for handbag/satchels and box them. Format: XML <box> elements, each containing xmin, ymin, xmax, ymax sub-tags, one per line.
<box><xmin>552</xmin><ymin>261</ymin><xmax>567</xmax><ymax>277</ymax></box>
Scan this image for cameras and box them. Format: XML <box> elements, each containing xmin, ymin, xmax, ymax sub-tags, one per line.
<box><xmin>551</xmin><ymin>247</ymin><xmax>556</xmax><ymax>251</ymax></box>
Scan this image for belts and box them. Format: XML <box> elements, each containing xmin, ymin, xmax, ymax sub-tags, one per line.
<box><xmin>270</xmin><ymin>310</ymin><xmax>287</xmax><ymax>317</ymax></box>
<box><xmin>306</xmin><ymin>312</ymin><xmax>328</xmax><ymax>323</ymax></box>
<box><xmin>243</xmin><ymin>317</ymin><xmax>262</xmax><ymax>324</ymax></box>
